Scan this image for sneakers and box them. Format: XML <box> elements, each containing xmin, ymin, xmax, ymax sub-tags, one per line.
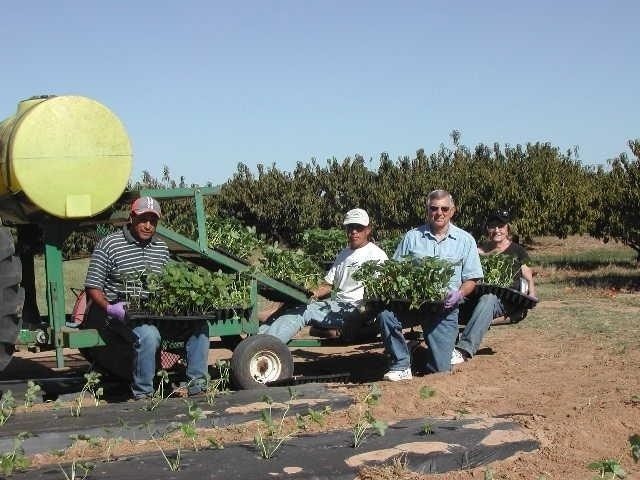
<box><xmin>382</xmin><ymin>367</ymin><xmax>412</xmax><ymax>381</ymax></box>
<box><xmin>448</xmin><ymin>347</ymin><xmax>469</xmax><ymax>365</ymax></box>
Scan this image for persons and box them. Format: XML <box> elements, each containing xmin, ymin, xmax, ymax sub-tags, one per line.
<box><xmin>376</xmin><ymin>190</ymin><xmax>483</xmax><ymax>382</ymax></box>
<box><xmin>85</xmin><ymin>196</ymin><xmax>210</xmax><ymax>401</ymax></box>
<box><xmin>450</xmin><ymin>207</ymin><xmax>535</xmax><ymax>365</ymax></box>
<box><xmin>256</xmin><ymin>209</ymin><xmax>389</xmax><ymax>344</ymax></box>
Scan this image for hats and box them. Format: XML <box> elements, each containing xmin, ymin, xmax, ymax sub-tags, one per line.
<box><xmin>131</xmin><ymin>196</ymin><xmax>162</xmax><ymax>219</ymax></box>
<box><xmin>342</xmin><ymin>207</ymin><xmax>370</xmax><ymax>227</ymax></box>
<box><xmin>486</xmin><ymin>208</ymin><xmax>512</xmax><ymax>224</ymax></box>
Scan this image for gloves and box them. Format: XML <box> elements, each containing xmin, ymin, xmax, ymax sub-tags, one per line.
<box><xmin>444</xmin><ymin>290</ymin><xmax>463</xmax><ymax>309</ymax></box>
<box><xmin>106</xmin><ymin>301</ymin><xmax>131</xmax><ymax>322</ymax></box>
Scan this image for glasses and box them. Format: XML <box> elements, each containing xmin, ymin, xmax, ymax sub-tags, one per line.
<box><xmin>429</xmin><ymin>205</ymin><xmax>454</xmax><ymax>212</ymax></box>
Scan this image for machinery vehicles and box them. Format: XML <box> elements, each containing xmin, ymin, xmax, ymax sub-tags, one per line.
<box><xmin>0</xmin><ymin>95</ymin><xmax>387</xmax><ymax>389</ymax></box>
